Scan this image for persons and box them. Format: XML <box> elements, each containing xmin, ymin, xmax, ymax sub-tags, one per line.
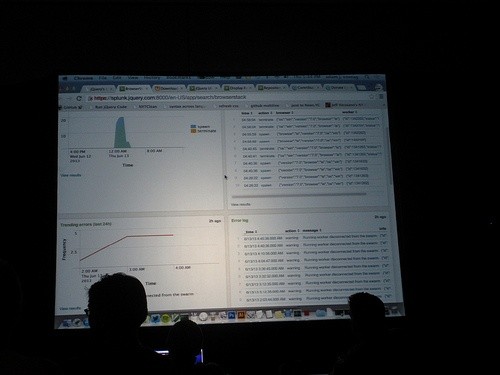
<box><xmin>52</xmin><ymin>273</ymin><xmax>159</xmax><ymax>375</ymax></box>
<box><xmin>332</xmin><ymin>291</ymin><xmax>408</xmax><ymax>363</ymax></box>
<box><xmin>155</xmin><ymin>317</ymin><xmax>222</xmax><ymax>375</ymax></box>
